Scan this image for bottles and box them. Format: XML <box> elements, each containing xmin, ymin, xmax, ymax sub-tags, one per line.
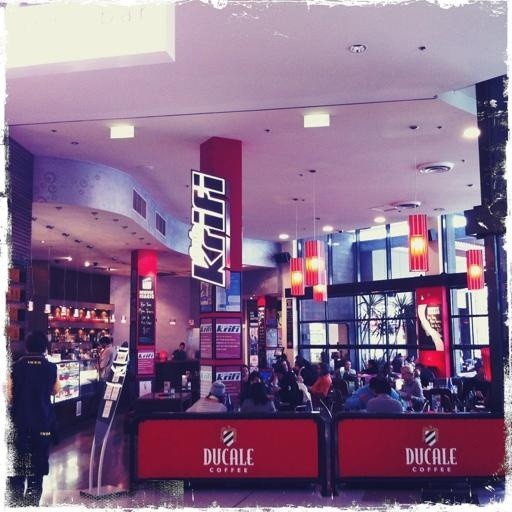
<box><xmin>223</xmin><ymin>393</ymin><xmax>232</xmax><ymax>412</ymax></box>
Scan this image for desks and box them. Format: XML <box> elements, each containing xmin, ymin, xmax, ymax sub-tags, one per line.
<box><xmin>123</xmin><ymin>390</ymin><xmax>200</xmax><ymax>442</ymax></box>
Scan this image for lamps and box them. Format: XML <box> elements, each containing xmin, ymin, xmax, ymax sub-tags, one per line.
<box><xmin>290</xmin><ymin>169</ymin><xmax>328</xmax><ymax>303</ymax></box>
<box><xmin>27</xmin><ymin>217</ymin><xmax>126</xmax><ymax>323</ymax></box>
<box><xmin>466</xmin><ymin>235</ymin><xmax>484</xmax><ymax>290</ymax></box>
<box><xmin>169</xmin><ymin>318</ymin><xmax>176</xmax><ymax>325</ymax></box>
<box><xmin>408</xmin><ymin>124</ymin><xmax>430</xmax><ymax>271</ymax></box>
<box><xmin>111</xmin><ymin>126</ymin><xmax>134</xmax><ymax>139</ymax></box>
<box><xmin>303</xmin><ymin>114</ymin><xmax>329</xmax><ymax>128</ymax></box>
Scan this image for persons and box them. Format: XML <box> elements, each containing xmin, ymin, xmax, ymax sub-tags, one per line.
<box><xmin>184</xmin><ymin>350</ymin><xmax>490</xmax><ymax>414</ymax></box>
<box><xmin>79</xmin><ymin>344</ymin><xmax>102</xmax><ymax>360</ymax></box>
<box><xmin>95</xmin><ymin>336</ymin><xmax>115</xmax><ymax>383</ymax></box>
<box><xmin>170</xmin><ymin>341</ymin><xmax>187</xmax><ymax>360</ymax></box>
<box><xmin>6</xmin><ymin>330</ymin><xmax>61</xmax><ymax>506</ymax></box>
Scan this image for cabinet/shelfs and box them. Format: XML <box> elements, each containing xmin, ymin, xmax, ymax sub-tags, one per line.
<box><xmin>49</xmin><ymin>360</ymin><xmax>80</xmax><ymax>406</ymax></box>
<box><xmin>47</xmin><ymin>321</ymin><xmax>114</xmax><ymax>351</ymax></box>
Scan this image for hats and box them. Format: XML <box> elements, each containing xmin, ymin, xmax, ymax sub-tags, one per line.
<box><xmin>209</xmin><ymin>381</ymin><xmax>226</xmax><ymax>397</ymax></box>
<box><xmin>460</xmin><ymin>358</ymin><xmax>483</xmax><ymax>372</ymax></box>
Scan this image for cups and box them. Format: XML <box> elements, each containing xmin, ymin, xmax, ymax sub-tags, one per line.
<box><xmin>429</xmin><ymin>382</ymin><xmax>433</xmax><ymax>388</ymax></box>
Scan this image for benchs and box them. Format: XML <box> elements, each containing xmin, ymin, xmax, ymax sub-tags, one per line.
<box><xmin>330</xmin><ymin>411</ymin><xmax>505</xmax><ymax>496</ymax></box>
<box><xmin>124</xmin><ymin>411</ymin><xmax>327</xmax><ymax>497</ymax></box>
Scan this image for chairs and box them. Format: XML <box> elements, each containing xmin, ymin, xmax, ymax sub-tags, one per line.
<box><xmin>226</xmin><ymin>357</ymin><xmax>492</xmax><ymax>418</ymax></box>
